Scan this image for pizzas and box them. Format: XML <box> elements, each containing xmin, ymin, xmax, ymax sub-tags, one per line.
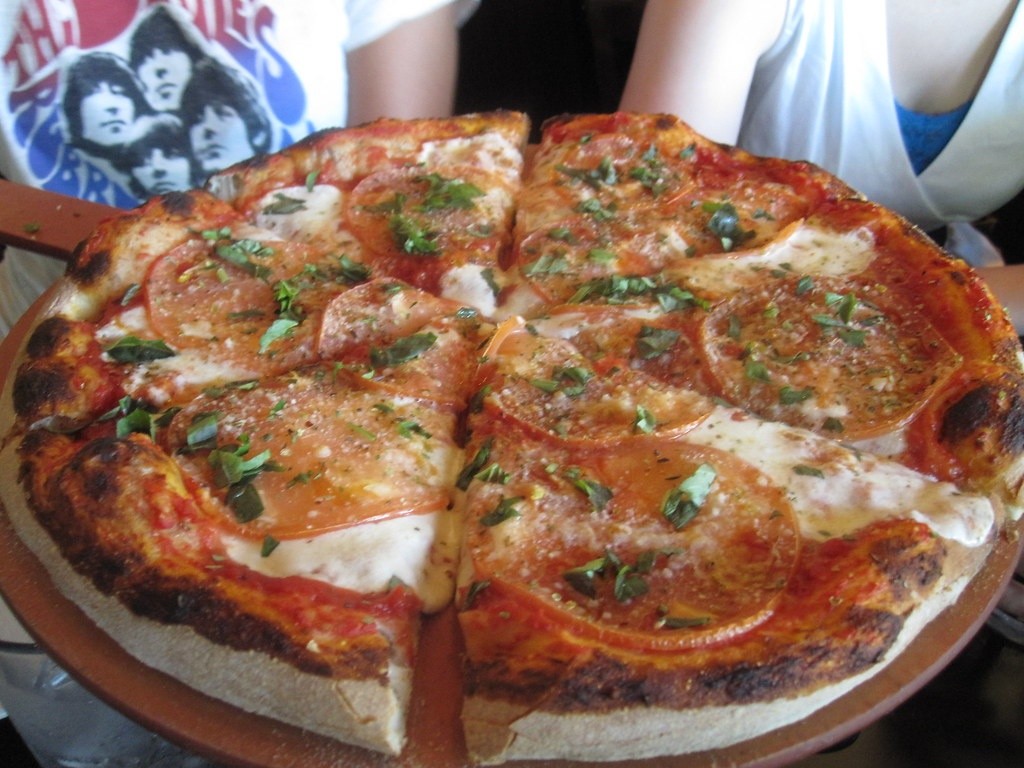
<box><xmin>1</xmin><ymin>109</ymin><xmax>1021</xmax><ymax>768</ymax></box>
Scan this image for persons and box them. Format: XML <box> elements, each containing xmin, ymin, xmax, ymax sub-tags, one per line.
<box><xmin>0</xmin><ymin>0</ymin><xmax>480</xmax><ymax>352</ymax></box>
<box><xmin>616</xmin><ymin>1</ymin><xmax>1024</xmax><ymax>342</ymax></box>
